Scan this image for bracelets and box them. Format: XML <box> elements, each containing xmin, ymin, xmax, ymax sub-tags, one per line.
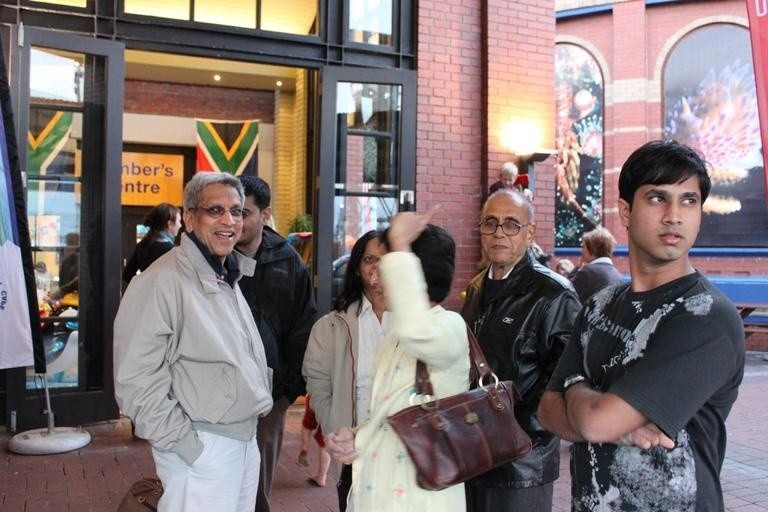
<box><xmin>616</xmin><ymin>430</ymin><xmax>634</xmax><ymax>447</ymax></box>
<box><xmin>563</xmin><ymin>373</ymin><xmax>588</xmax><ymax>393</ymax></box>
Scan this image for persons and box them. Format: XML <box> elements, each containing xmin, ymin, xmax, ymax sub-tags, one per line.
<box><xmin>234</xmin><ymin>175</ymin><xmax>318</xmax><ymax>511</ymax></box>
<box><xmin>478</xmin><ymin>188</ymin><xmax>552</xmax><ymax>274</ymax></box>
<box><xmin>325</xmin><ymin>202</ymin><xmax>473</xmax><ymax>511</ymax></box>
<box><xmin>301</xmin><ymin>230</ymin><xmax>387</xmax><ymax>511</ymax></box>
<box><xmin>490</xmin><ymin>162</ymin><xmax>519</xmax><ymax>193</ymax></box>
<box><xmin>458</xmin><ymin>189</ymin><xmax>584</xmax><ymax>511</ymax></box>
<box><xmin>60</xmin><ymin>232</ymin><xmax>79</xmax><ymax>296</ymax></box>
<box><xmin>109</xmin><ymin>172</ymin><xmax>277</xmax><ymax>510</ymax></box>
<box><xmin>122</xmin><ymin>202</ymin><xmax>183</xmax><ymax>440</ymax></box>
<box><xmin>298</xmin><ymin>394</ymin><xmax>331</xmax><ymax>486</ymax></box>
<box><xmin>568</xmin><ymin>226</ymin><xmax>625</xmax><ymax>306</ymax></box>
<box><xmin>555</xmin><ymin>256</ymin><xmax>577</xmax><ymax>277</ymax></box>
<box><xmin>536</xmin><ymin>139</ymin><xmax>747</xmax><ymax>511</ymax></box>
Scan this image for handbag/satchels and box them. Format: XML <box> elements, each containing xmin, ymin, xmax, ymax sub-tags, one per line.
<box><xmin>385</xmin><ymin>381</ymin><xmax>532</xmax><ymax>491</ymax></box>
<box><xmin>116</xmin><ymin>478</ymin><xmax>164</xmax><ymax>512</ymax></box>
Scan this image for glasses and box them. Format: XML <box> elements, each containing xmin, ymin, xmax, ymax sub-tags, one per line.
<box><xmin>477</xmin><ymin>221</ymin><xmax>531</xmax><ymax>236</ymax></box>
<box><xmin>188</xmin><ymin>205</ymin><xmax>242</xmax><ymax>218</ymax></box>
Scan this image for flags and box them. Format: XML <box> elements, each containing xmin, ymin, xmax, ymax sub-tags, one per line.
<box><xmin>196</xmin><ymin>118</ymin><xmax>264</xmax><ymax>184</ymax></box>
<box><xmin>25</xmin><ymin>103</ymin><xmax>74</xmax><ymax>193</ymax></box>
<box><xmin>0</xmin><ymin>59</ymin><xmax>46</xmax><ymax>376</ymax></box>
